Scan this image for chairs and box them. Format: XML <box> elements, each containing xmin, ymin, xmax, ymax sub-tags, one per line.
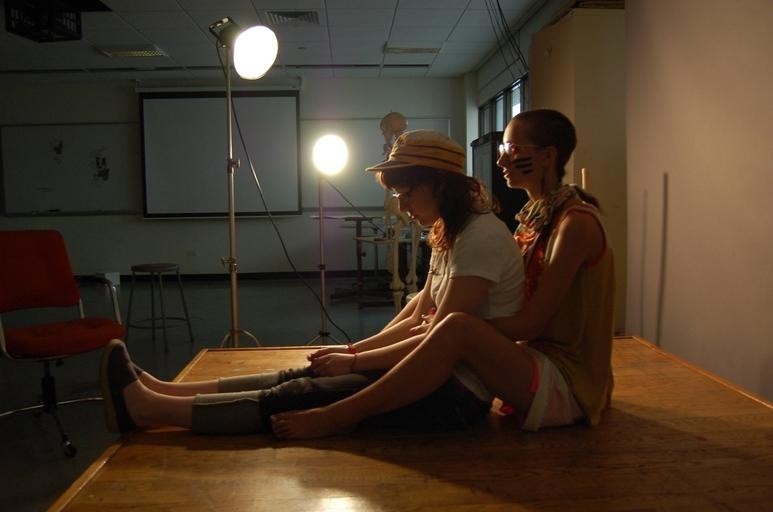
<box><xmin>0</xmin><ymin>228</ymin><xmax>129</xmax><ymax>458</ymax></box>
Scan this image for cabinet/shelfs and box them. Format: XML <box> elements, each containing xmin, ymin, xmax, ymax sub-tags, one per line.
<box><xmin>470</xmin><ymin>130</ymin><xmax>529</xmax><ymax>235</ymax></box>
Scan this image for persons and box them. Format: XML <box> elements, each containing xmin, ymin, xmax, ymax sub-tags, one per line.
<box><xmin>269</xmin><ymin>108</ymin><xmax>616</xmax><ymax>442</ymax></box>
<box><xmin>95</xmin><ymin>127</ymin><xmax>525</xmax><ymax>436</ymax></box>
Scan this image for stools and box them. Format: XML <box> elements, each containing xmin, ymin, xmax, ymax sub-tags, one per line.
<box><xmin>123</xmin><ymin>263</ymin><xmax>195</xmax><ymax>352</ymax></box>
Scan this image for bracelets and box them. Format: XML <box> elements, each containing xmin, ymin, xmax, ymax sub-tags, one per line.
<box><xmin>350</xmin><ymin>353</ymin><xmax>357</xmax><ymax>372</ymax></box>
<box><xmin>347</xmin><ymin>344</ymin><xmax>352</xmax><ymax>352</ymax></box>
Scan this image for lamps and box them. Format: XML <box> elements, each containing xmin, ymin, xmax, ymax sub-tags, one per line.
<box><xmin>207</xmin><ymin>15</ymin><xmax>280</xmax><ymax>349</ymax></box>
<box><xmin>303</xmin><ymin>134</ymin><xmax>345</xmax><ymax>345</ymax></box>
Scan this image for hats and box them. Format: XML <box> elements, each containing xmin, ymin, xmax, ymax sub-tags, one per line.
<box><xmin>366</xmin><ymin>128</ymin><xmax>465</xmax><ymax>175</ymax></box>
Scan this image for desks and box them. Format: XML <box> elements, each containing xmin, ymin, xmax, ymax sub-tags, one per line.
<box><xmin>310</xmin><ymin>215</ymin><xmax>392</xmax><ymax>305</ymax></box>
<box><xmin>353</xmin><ymin>234</ymin><xmax>425</xmax><ymax>309</ymax></box>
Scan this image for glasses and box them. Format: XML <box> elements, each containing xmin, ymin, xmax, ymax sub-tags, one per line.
<box><xmin>497</xmin><ymin>142</ymin><xmax>544</xmax><ymax>156</ymax></box>
<box><xmin>391</xmin><ymin>186</ymin><xmax>416</xmax><ymax>203</ymax></box>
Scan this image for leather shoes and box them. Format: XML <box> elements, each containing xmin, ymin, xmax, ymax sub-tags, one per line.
<box><xmin>130</xmin><ymin>360</ymin><xmax>145</xmax><ymax>377</ymax></box>
<box><xmin>99</xmin><ymin>339</ymin><xmax>139</xmax><ymax>435</ymax></box>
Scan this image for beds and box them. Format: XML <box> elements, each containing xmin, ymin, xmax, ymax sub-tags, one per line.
<box><xmin>46</xmin><ymin>335</ymin><xmax>773</xmax><ymax>512</ymax></box>
